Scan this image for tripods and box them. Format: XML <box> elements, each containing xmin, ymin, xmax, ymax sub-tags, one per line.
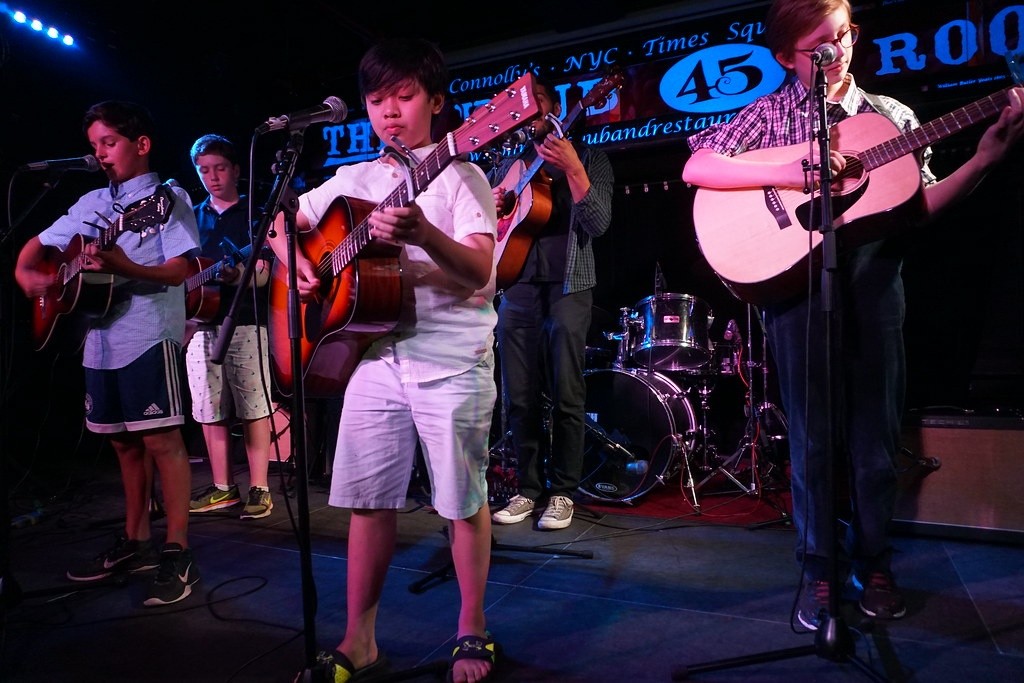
<box><xmin>406</xmin><ymin>534</ymin><xmax>594</xmax><ymax>593</ymax></box>
<box><xmin>679</xmin><ymin>303</ymin><xmax>789</xmax><ymax>519</ymax></box>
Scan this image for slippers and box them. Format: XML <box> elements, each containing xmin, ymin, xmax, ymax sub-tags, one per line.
<box><xmin>291</xmin><ymin>647</ymin><xmax>382</xmax><ymax>683</ymax></box>
<box><xmin>445</xmin><ymin>631</ymin><xmax>495</xmax><ymax>683</ymax></box>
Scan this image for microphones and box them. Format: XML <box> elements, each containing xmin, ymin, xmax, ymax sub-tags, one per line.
<box><xmin>724</xmin><ymin>319</ymin><xmax>742</xmax><ymax>344</ymax></box>
<box><xmin>811</xmin><ymin>44</ymin><xmax>838</xmax><ymax>66</ymax></box>
<box><xmin>256</xmin><ymin>96</ymin><xmax>348</xmax><ymax>136</ymax></box>
<box><xmin>627</xmin><ymin>460</ymin><xmax>648</xmax><ymax>478</ymax></box>
<box><xmin>508</xmin><ymin>132</ymin><xmax>527</xmax><ymax>144</ymax></box>
<box><xmin>656</xmin><ymin>262</ymin><xmax>668</xmax><ymax>291</ymax></box>
<box><xmin>916</xmin><ymin>455</ymin><xmax>941</xmax><ymax>468</ymax></box>
<box><xmin>20</xmin><ymin>155</ymin><xmax>99</xmax><ymax>173</ymax></box>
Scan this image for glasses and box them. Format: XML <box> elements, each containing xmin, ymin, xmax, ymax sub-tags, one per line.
<box><xmin>794</xmin><ymin>22</ymin><xmax>859</xmax><ymax>60</ymax></box>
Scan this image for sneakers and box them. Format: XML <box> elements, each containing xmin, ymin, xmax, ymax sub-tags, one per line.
<box><xmin>67</xmin><ymin>533</ymin><xmax>158</xmax><ymax>580</ymax></box>
<box><xmin>492</xmin><ymin>493</ymin><xmax>535</xmax><ymax>523</ymax></box>
<box><xmin>798</xmin><ymin>579</ymin><xmax>830</xmax><ymax>629</ymax></box>
<box><xmin>189</xmin><ymin>483</ymin><xmax>241</xmax><ymax>512</ymax></box>
<box><xmin>858</xmin><ymin>570</ymin><xmax>908</xmax><ymax>617</ymax></box>
<box><xmin>239</xmin><ymin>487</ymin><xmax>274</xmax><ymax>519</ymax></box>
<box><xmin>144</xmin><ymin>543</ymin><xmax>200</xmax><ymax>606</ymax></box>
<box><xmin>538</xmin><ymin>495</ymin><xmax>575</xmax><ymax>529</ymax></box>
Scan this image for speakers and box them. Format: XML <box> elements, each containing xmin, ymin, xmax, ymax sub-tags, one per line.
<box><xmin>265</xmin><ymin>403</ymin><xmax>315</xmax><ymax>468</ymax></box>
<box><xmin>887</xmin><ymin>414</ymin><xmax>1024</xmax><ymax>544</ymax></box>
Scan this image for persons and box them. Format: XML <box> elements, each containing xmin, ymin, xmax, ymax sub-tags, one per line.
<box><xmin>486</xmin><ymin>78</ymin><xmax>614</xmax><ymax>530</ymax></box>
<box><xmin>186</xmin><ymin>135</ymin><xmax>276</xmax><ymax>519</ymax></box>
<box><xmin>15</xmin><ymin>102</ymin><xmax>201</xmax><ymax>606</ymax></box>
<box><xmin>681</xmin><ymin>0</ymin><xmax>1024</xmax><ymax>632</ymax></box>
<box><xmin>266</xmin><ymin>35</ymin><xmax>497</xmax><ymax>683</ymax></box>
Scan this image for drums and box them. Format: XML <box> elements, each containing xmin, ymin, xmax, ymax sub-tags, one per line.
<box><xmin>549</xmin><ymin>368</ymin><xmax>697</xmax><ymax>502</ymax></box>
<box><xmin>630</xmin><ymin>291</ymin><xmax>713</xmax><ymax>372</ymax></box>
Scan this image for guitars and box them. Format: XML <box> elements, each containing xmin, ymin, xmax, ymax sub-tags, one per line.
<box><xmin>490</xmin><ymin>62</ymin><xmax>629</xmax><ymax>293</ymax></box>
<box><xmin>181</xmin><ymin>243</ymin><xmax>256</xmax><ymax>347</ymax></box>
<box><xmin>27</xmin><ymin>182</ymin><xmax>176</xmax><ymax>356</ymax></box>
<box><xmin>265</xmin><ymin>68</ymin><xmax>546</xmax><ymax>405</ymax></box>
<box><xmin>691</xmin><ymin>82</ymin><xmax>1023</xmax><ymax>307</ymax></box>
